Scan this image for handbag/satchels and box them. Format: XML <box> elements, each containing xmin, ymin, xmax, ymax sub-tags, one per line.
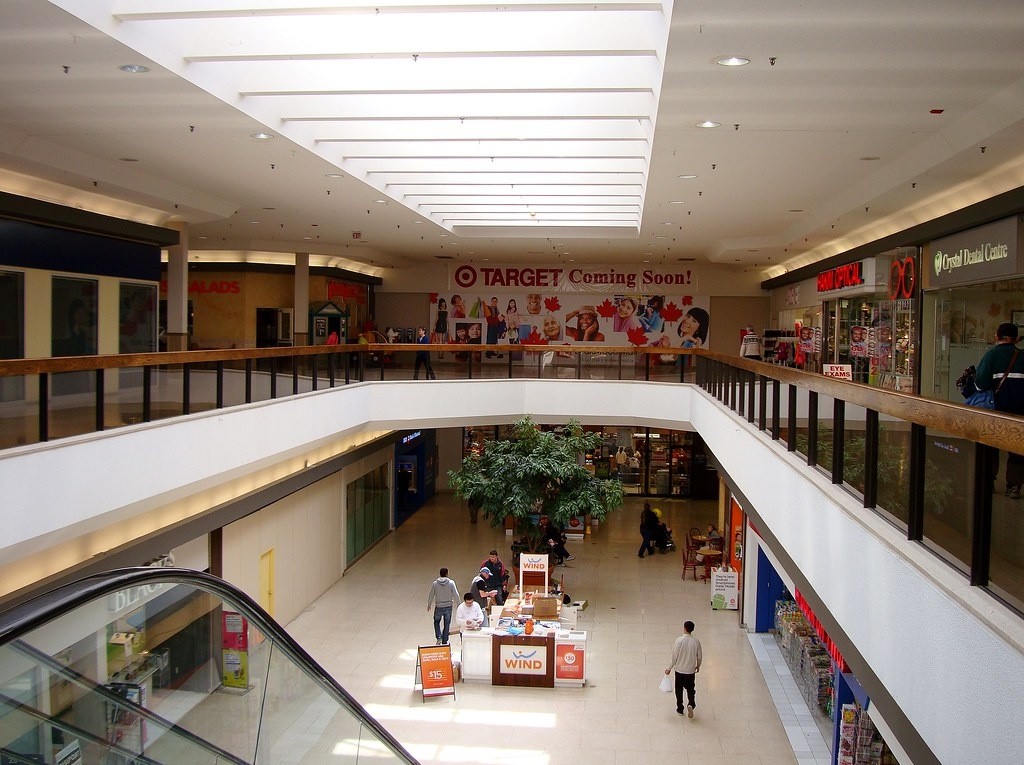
<box><xmin>659</xmin><ymin>675</ymin><xmax>673</xmax><ymax>693</ymax></box>
<box><xmin>964</xmin><ymin>390</ymin><xmax>997</xmax><ymax>409</ymax></box>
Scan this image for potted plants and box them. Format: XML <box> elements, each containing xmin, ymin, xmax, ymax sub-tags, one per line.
<box><xmin>447</xmin><ymin>412</ymin><xmax>628</xmax><ymax>586</ymax></box>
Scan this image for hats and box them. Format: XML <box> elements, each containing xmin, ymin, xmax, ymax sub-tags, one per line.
<box><xmin>480</xmin><ymin>567</ymin><xmax>493</xmax><ymax>576</ymax></box>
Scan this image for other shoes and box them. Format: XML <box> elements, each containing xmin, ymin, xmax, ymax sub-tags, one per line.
<box><xmin>638</xmin><ymin>554</ymin><xmax>644</xmax><ymax>558</ymax></box>
<box><xmin>648</xmin><ymin>550</ymin><xmax>654</xmax><ymax>554</ymax></box>
<box><xmin>471</xmin><ymin>520</ymin><xmax>477</xmax><ymax>524</ymax></box>
<box><xmin>676</xmin><ymin>709</ymin><xmax>683</xmax><ymax>716</ymax></box>
<box><xmin>557</xmin><ymin>563</ymin><xmax>566</xmax><ymax>566</ymax></box>
<box><xmin>688</xmin><ymin>705</ymin><xmax>693</xmax><ymax>718</ymax></box>
<box><xmin>566</xmin><ymin>556</ymin><xmax>574</xmax><ymax>560</ymax></box>
<box><xmin>436</xmin><ymin>639</ymin><xmax>442</xmax><ymax>645</ymax></box>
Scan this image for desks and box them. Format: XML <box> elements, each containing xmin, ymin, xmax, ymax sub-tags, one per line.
<box><xmin>499</xmin><ymin>584</ymin><xmax>563</xmax><ymax>621</ymax></box>
<box><xmin>695</xmin><ymin>549</ymin><xmax>722</xmax><ymax>584</ymax></box>
<box><xmin>691</xmin><ymin>535</ymin><xmax>708</xmax><ymax>550</ymax></box>
<box><xmin>460</xmin><ymin>627</ymin><xmax>588</xmax><ymax>687</ymax></box>
<box><xmin>109</xmin><ymin>652</ymin><xmax>162</xmax><ymax>698</ymax></box>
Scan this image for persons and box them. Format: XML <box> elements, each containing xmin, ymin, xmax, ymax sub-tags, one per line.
<box><xmin>456</xmin><ymin>593</ymin><xmax>484</xmax><ymax>662</ymax></box>
<box><xmin>800</xmin><ymin>327</ymin><xmax>814</xmax><ymax>341</ymax></box>
<box><xmin>470</xmin><ymin>549</ymin><xmax>506</xmax><ymax>627</ymax></box>
<box><xmin>426</xmin><ymin>568</ymin><xmax>461</xmax><ymax>645</ymax></box>
<box><xmin>739</xmin><ymin>325</ymin><xmax>762</xmax><ymax>361</ymax></box>
<box><xmin>665</xmin><ymin>621</ymin><xmax>702</xmax><ymax>718</ymax></box>
<box><xmin>692</xmin><ymin>524</ymin><xmax>720</xmax><ymax>565</ymax></box>
<box><xmin>851</xmin><ymin>327</ymin><xmax>867</xmax><ymax>344</ymax></box>
<box><xmin>511</xmin><ymin>515</ymin><xmax>575</xmax><ymax>567</ymax></box>
<box><xmin>433</xmin><ymin>294</ymin><xmax>709</xmax><ymax>367</ymax></box>
<box><xmin>413</xmin><ymin>326</ymin><xmax>436</xmax><ymax>380</ymax></box>
<box><xmin>638</xmin><ymin>503</ymin><xmax>660</xmax><ymax>558</ymax></box>
<box><xmin>468</xmin><ymin>492</ymin><xmax>479</xmax><ymax>524</ymax></box>
<box><xmin>974</xmin><ymin>323</ymin><xmax>1024</xmax><ymax>499</ymax></box>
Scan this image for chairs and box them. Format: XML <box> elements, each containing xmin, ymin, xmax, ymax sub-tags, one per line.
<box><xmin>682</xmin><ymin>547</ymin><xmax>697</xmax><ymax>582</ymax></box>
<box><xmin>690</xmin><ymin>528</ymin><xmax>705</xmax><ymax>556</ymax></box>
<box><xmin>709</xmin><ymin>536</ymin><xmax>723</xmax><ymax>571</ymax></box>
<box><xmin>686</xmin><ymin>533</ymin><xmax>700</xmax><ymax>561</ymax></box>
<box><xmin>717</xmin><ymin>537</ymin><xmax>725</xmax><ymax>557</ymax></box>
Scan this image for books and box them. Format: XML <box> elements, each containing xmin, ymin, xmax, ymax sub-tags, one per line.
<box><xmin>837</xmin><ymin>704</ymin><xmax>856</xmax><ymax>765</ymax></box>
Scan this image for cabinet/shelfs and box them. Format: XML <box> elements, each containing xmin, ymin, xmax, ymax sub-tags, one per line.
<box><xmin>762</xmin><ymin>328</ymin><xmax>796</xmax><ymax>369</ymax></box>
<box><xmin>949</xmin><ymin>342</ymin><xmax>994</xmax><ymax>404</ymax></box>
<box><xmin>774</xmin><ymin>600</ymin><xmax>832</xmax><ymax>714</ymax></box>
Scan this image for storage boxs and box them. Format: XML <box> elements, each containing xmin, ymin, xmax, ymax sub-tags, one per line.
<box><xmin>452</xmin><ymin>661</ymin><xmax>462</xmax><ymax>683</ymax></box>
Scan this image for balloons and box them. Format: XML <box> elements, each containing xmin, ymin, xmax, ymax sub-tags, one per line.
<box><xmin>652</xmin><ymin>508</ymin><xmax>662</xmax><ymax>522</ymax></box>
